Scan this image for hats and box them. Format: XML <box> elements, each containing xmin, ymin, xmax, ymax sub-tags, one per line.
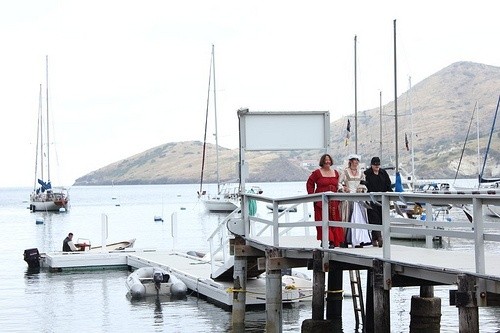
<box><xmin>371</xmin><ymin>157</ymin><xmax>380</xmax><ymax>165</ymax></box>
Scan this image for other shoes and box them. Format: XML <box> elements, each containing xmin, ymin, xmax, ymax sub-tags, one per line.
<box><xmin>377</xmin><ymin>238</ymin><xmax>383</xmax><ymax>247</ymax></box>
<box><xmin>329</xmin><ymin>244</ymin><xmax>335</xmax><ymax>248</ymax></box>
<box><xmin>372</xmin><ymin>239</ymin><xmax>378</xmax><ymax>247</ymax></box>
<box><xmin>340</xmin><ymin>242</ymin><xmax>348</xmax><ymax>248</ymax></box>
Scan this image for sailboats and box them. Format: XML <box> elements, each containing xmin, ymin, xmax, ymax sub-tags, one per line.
<box><xmin>31</xmin><ymin>82</ymin><xmax>69</xmax><ymax>213</ymax></box>
<box><xmin>347</xmin><ymin>19</ymin><xmax>500</xmax><ymax>223</ymax></box>
<box><xmin>197</xmin><ymin>43</ymin><xmax>262</xmax><ymax>213</ymax></box>
<box><xmin>23</xmin><ymin>55</ymin><xmax>135</xmax><ymax>254</ymax></box>
